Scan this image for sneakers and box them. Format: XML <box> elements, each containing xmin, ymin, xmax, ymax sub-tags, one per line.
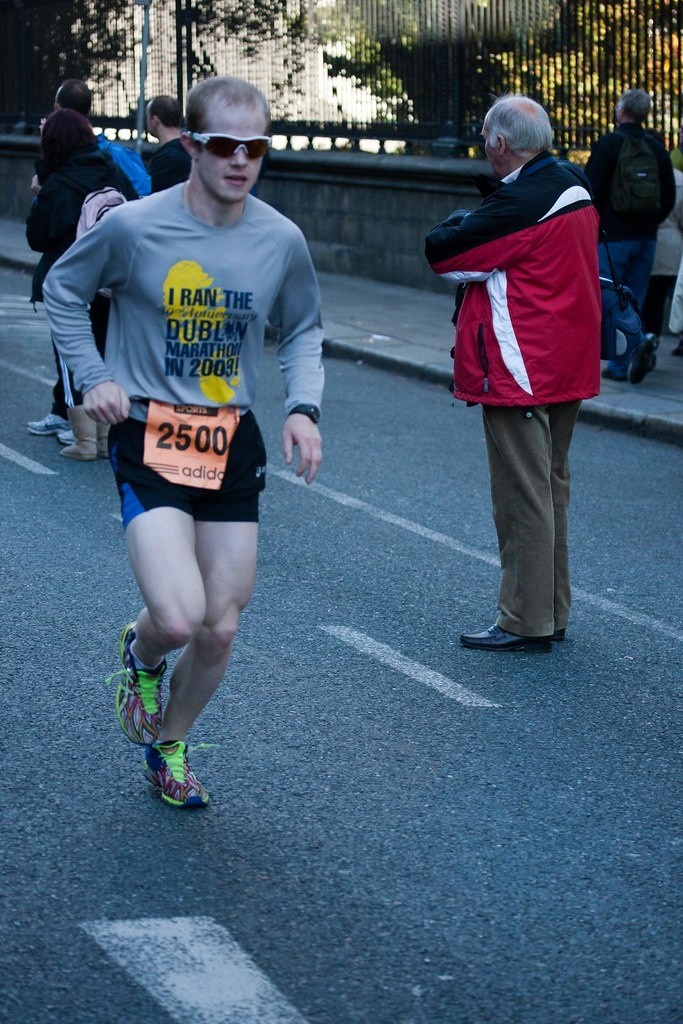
<box><xmin>57</xmin><ymin>430</ymin><xmax>76</xmax><ymax>445</ymax></box>
<box><xmin>26</xmin><ymin>414</ymin><xmax>71</xmax><ymax>435</ymax></box>
<box><xmin>105</xmin><ymin>621</ymin><xmax>167</xmax><ymax>746</ymax></box>
<box><xmin>143</xmin><ymin>738</ymin><xmax>222</xmax><ymax>808</ymax></box>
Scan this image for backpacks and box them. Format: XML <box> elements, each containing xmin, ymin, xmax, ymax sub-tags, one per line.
<box><xmin>608</xmin><ymin>130</ymin><xmax>662</xmax><ymax>217</ymax></box>
<box><xmin>54</xmin><ymin>174</ymin><xmax>128</xmax><ymax>298</ymax></box>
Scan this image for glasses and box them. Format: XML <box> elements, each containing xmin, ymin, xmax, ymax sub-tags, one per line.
<box><xmin>187</xmin><ymin>131</ymin><xmax>272</xmax><ymax>159</ymax></box>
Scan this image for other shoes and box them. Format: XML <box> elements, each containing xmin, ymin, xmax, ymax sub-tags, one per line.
<box><xmin>602</xmin><ymin>369</ymin><xmax>628</xmax><ymax>382</ymax></box>
<box><xmin>627</xmin><ymin>333</ymin><xmax>655</xmax><ymax>384</ymax></box>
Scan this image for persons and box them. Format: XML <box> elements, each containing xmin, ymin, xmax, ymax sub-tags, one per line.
<box><xmin>25</xmin><ymin>79</ymin><xmax>192</xmax><ymax>461</ymax></box>
<box><xmin>43</xmin><ymin>77</ymin><xmax>326</xmax><ymax>807</ymax></box>
<box><xmin>583</xmin><ymin>89</ymin><xmax>676</xmax><ymax>385</ymax></box>
<box><xmin>423</xmin><ymin>96</ymin><xmax>603</xmax><ymax>653</ymax></box>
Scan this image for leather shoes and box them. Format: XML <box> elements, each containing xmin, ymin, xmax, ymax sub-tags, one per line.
<box><xmin>551</xmin><ymin>628</ymin><xmax>565</xmax><ymax>641</ymax></box>
<box><xmin>458</xmin><ymin>623</ymin><xmax>551</xmax><ymax>653</ymax></box>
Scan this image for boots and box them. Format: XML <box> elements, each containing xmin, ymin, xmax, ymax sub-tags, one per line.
<box><xmin>60</xmin><ymin>405</ymin><xmax>97</xmax><ymax>461</ymax></box>
<box><xmin>96</xmin><ymin>422</ymin><xmax>110</xmax><ymax>458</ymax></box>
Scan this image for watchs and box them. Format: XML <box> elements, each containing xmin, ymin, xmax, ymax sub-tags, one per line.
<box><xmin>289</xmin><ymin>403</ymin><xmax>321</xmax><ymax>424</ymax></box>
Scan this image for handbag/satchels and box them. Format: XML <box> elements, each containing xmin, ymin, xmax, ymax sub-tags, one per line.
<box><xmin>599</xmin><ymin>276</ymin><xmax>643</xmax><ymax>359</ymax></box>
